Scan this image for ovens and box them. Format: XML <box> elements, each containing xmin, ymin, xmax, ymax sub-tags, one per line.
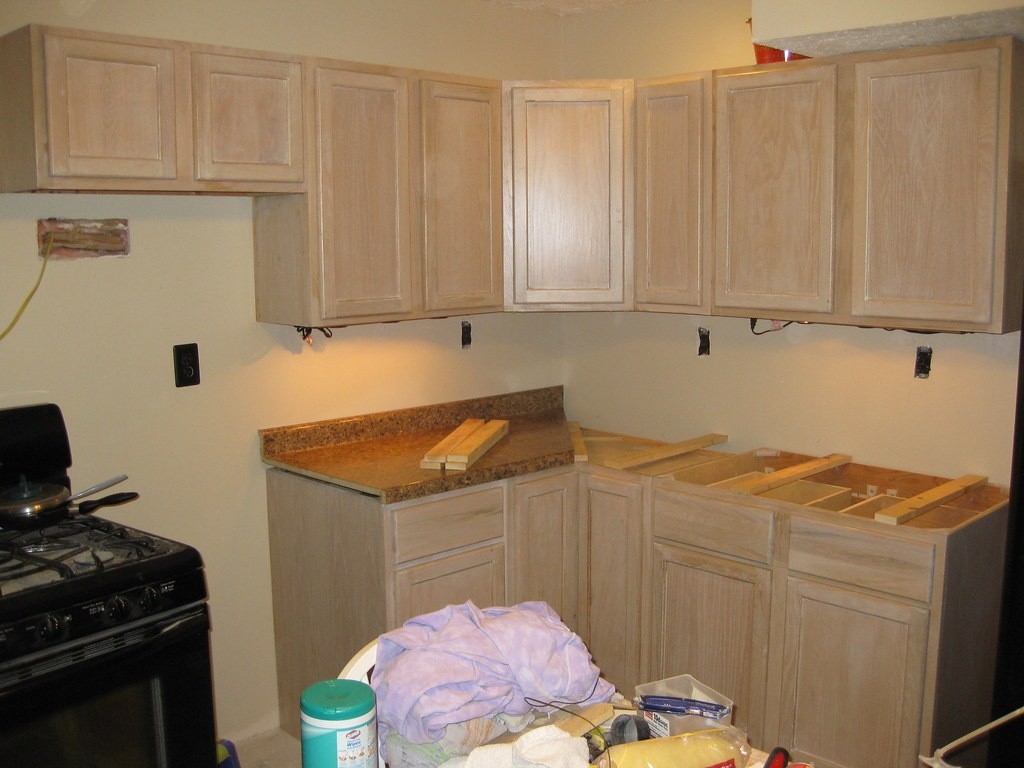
<box><xmin>0</xmin><ymin>600</ymin><xmax>218</xmax><ymax>768</ymax></box>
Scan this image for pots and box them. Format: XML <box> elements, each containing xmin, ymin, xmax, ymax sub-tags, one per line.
<box><xmin>0</xmin><ymin>476</ymin><xmax>138</xmax><ymax>531</ymax></box>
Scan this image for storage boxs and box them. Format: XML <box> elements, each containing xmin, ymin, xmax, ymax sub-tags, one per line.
<box><xmin>631</xmin><ymin>675</ymin><xmax>734</xmax><ymax>739</ymax></box>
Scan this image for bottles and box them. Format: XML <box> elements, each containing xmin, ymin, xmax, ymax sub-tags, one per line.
<box><xmin>300</xmin><ymin>679</ymin><xmax>378</xmax><ymax>768</ymax></box>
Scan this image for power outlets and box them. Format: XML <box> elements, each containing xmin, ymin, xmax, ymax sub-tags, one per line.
<box><xmin>174</xmin><ymin>344</ymin><xmax>200</xmax><ymax>387</ymax></box>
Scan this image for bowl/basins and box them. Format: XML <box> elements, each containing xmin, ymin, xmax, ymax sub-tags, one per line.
<box><xmin>634</xmin><ymin>673</ymin><xmax>733</xmax><ymax>738</ymax></box>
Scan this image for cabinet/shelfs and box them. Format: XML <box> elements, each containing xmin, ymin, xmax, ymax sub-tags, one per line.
<box><xmin>263</xmin><ymin>466</ymin><xmax>1010</xmax><ymax>767</ymax></box>
<box><xmin>0</xmin><ymin>35</ymin><xmax>1024</xmax><ymax>335</ymax></box>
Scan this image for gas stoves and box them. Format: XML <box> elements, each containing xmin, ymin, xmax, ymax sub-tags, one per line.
<box><xmin>0</xmin><ymin>402</ymin><xmax>210</xmax><ymax>650</ymax></box>
